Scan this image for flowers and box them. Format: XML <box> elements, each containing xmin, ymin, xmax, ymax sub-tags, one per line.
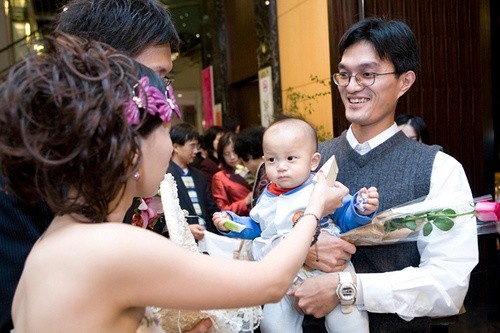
<box><xmin>131</xmin><ymin>197</ymin><xmax>158</xmax><ymax>230</ymax></box>
<box><xmin>384</xmin><ymin>199</ymin><xmax>500</xmax><ymax>237</ymax></box>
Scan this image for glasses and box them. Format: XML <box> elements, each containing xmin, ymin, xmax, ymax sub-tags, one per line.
<box><xmin>333</xmin><ymin>72</ymin><xmax>396</xmax><ymax>87</ymax></box>
<box><xmin>162</xmin><ymin>75</ymin><xmax>175</xmax><ymax>87</ymax></box>
<box><xmin>185</xmin><ymin>144</ymin><xmax>201</xmax><ymax>149</ymax></box>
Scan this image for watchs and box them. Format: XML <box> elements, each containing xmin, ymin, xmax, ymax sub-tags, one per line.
<box><xmin>336</xmin><ymin>272</ymin><xmax>358</xmax><ymax>314</ymax></box>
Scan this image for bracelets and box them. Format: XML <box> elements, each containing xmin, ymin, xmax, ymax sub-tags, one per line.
<box><xmin>292</xmin><ymin>214</ymin><xmax>321</xmax><ymax>247</ymax></box>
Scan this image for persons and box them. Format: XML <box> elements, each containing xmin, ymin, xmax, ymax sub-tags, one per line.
<box><xmin>0</xmin><ymin>0</ymin><xmax>479</xmax><ymax>333</ymax></box>
<box><xmin>10</xmin><ymin>32</ymin><xmax>350</xmax><ymax>333</ymax></box>
